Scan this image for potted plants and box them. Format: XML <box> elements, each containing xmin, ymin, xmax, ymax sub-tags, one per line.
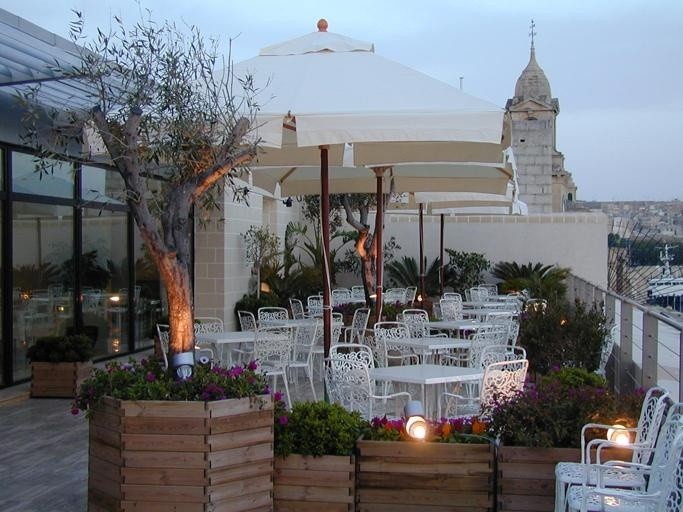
<box><xmin>26</xmin><ymin>333</ymin><xmax>94</xmax><ymax>398</ymax></box>
<box><xmin>484</xmin><ymin>364</ymin><xmax>645</xmax><ymax>512</ymax></box>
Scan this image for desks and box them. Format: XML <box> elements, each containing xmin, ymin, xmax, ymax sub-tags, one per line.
<box><xmin>367</xmin><ymin>362</ymin><xmax>498</xmax><ymax>423</ymax></box>
<box><xmin>193</xmin><ymin>331</ymin><xmax>281</xmax><ymax>369</ymax></box>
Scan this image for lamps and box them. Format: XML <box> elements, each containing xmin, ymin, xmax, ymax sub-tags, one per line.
<box><xmin>607</xmin><ymin>424</ymin><xmax>629</xmax><ymax>442</ymax></box>
<box><xmin>404</xmin><ymin>401</ymin><xmax>429</xmax><ymax>439</ymax></box>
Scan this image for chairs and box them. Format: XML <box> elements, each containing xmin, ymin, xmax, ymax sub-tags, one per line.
<box><xmin>157</xmin><ymin>324</ymin><xmax>214</xmax><ymax>371</ymax></box>
<box><xmin>232</xmin><ymin>325</ymin><xmax>294</xmax><ymax>413</ymax></box>
<box><xmin>288</xmin><ymin>317</ymin><xmax>319</xmax><ymax>404</ymax></box>
<box><xmin>239</xmin><ymin>285</ymin><xmax>527</xmax><ymax>367</ymax></box>
<box><xmin>13</xmin><ymin>285</ymin><xmax>141</xmax><ymax>341</ymax></box>
<box><xmin>323</xmin><ymin>359</ymin><xmax>412</xmax><ymax>422</ymax></box>
<box><xmin>555</xmin><ymin>386</ymin><xmax>669</xmax><ymax>512</ymax></box>
<box><xmin>437</xmin><ymin>359</ymin><xmax>530</xmax><ymax>423</ymax></box>
<box><xmin>329</xmin><ymin>343</ymin><xmax>377</xmax><ymax>395</ymax></box>
<box><xmin>567</xmin><ymin>403</ymin><xmax>683</xmax><ymax>512</ymax></box>
<box><xmin>194</xmin><ymin>317</ymin><xmax>224</xmax><ymax>360</ymax></box>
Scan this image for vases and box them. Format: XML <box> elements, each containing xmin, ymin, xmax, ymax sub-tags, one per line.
<box><xmin>88</xmin><ymin>394</ymin><xmax>275</xmax><ymax>512</ymax></box>
<box><xmin>355</xmin><ymin>434</ymin><xmax>495</xmax><ymax>512</ymax></box>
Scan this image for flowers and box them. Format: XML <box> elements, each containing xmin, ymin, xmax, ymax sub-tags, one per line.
<box><xmin>274</xmin><ymin>400</ymin><xmax>369</xmax><ymax>512</ymax></box>
<box><xmin>362</xmin><ymin>411</ymin><xmax>495</xmax><ymax>444</ymax></box>
<box><xmin>70</xmin><ymin>358</ymin><xmax>292</xmax><ymax>428</ymax></box>
